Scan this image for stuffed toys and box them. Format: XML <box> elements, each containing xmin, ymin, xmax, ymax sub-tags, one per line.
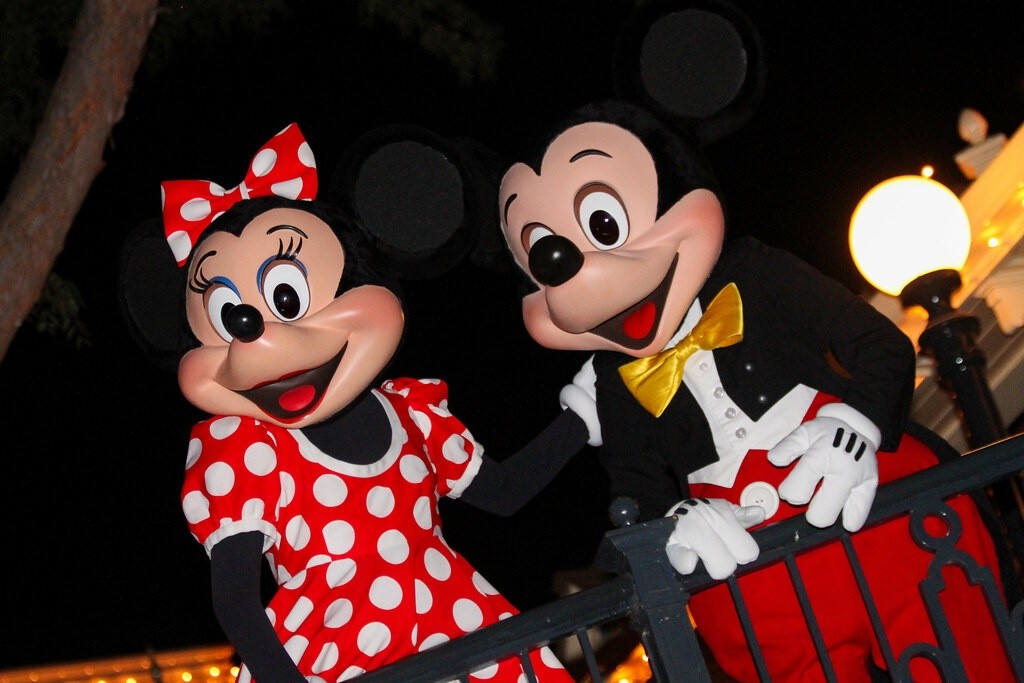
<box><xmin>451</xmin><ymin>7</ymin><xmax>1024</xmax><ymax>683</ymax></box>
<box><xmin>156</xmin><ymin>122</ymin><xmax>604</xmax><ymax>683</ymax></box>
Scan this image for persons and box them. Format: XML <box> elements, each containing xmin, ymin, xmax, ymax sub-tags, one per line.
<box><xmin>539</xmin><ymin>573</ymin><xmax>603</xmax><ymax>664</ymax></box>
